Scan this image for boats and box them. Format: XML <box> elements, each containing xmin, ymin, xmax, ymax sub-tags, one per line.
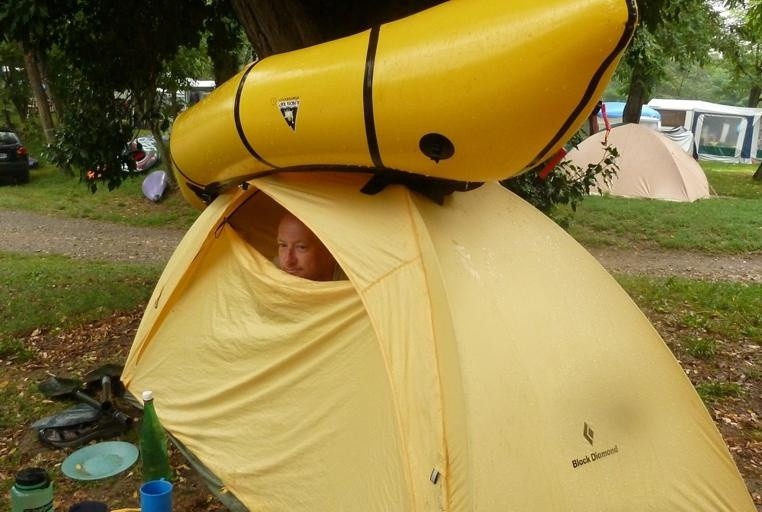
<box><xmin>140</xmin><ymin>171</ymin><xmax>168</xmax><ymax>203</ymax></box>
<box><xmin>167</xmin><ymin>0</ymin><xmax>642</xmax><ymax>213</ymax></box>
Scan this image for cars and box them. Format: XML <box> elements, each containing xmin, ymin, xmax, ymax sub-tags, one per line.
<box><xmin>0</xmin><ymin>130</ymin><xmax>30</xmax><ymax>186</ymax></box>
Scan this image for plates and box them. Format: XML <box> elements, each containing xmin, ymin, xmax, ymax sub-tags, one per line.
<box><xmin>61</xmin><ymin>441</ymin><xmax>139</xmax><ymax>481</ymax></box>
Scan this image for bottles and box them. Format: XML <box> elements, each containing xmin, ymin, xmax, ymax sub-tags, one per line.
<box><xmin>136</xmin><ymin>389</ymin><xmax>172</xmax><ymax>480</ymax></box>
<box><xmin>10</xmin><ymin>467</ymin><xmax>54</xmax><ymax>511</ymax></box>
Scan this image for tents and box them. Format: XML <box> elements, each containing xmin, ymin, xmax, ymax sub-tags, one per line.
<box><xmin>117</xmin><ymin>169</ymin><xmax>758</xmax><ymax>512</ymax></box>
<box><xmin>556</xmin><ymin>97</ymin><xmax>762</xmax><ymax>205</ymax></box>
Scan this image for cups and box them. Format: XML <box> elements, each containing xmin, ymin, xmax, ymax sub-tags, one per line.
<box><xmin>67</xmin><ymin>499</ymin><xmax>110</xmax><ymax>511</ymax></box>
<box><xmin>140</xmin><ymin>480</ymin><xmax>173</xmax><ymax>512</ymax></box>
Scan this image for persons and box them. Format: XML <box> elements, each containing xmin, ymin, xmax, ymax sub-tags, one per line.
<box><xmin>271</xmin><ymin>210</ymin><xmax>336</xmax><ymax>284</ymax></box>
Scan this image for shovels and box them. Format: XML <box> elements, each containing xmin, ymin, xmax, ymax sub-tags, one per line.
<box><xmin>30</xmin><ymin>364</ymin><xmax>133</xmax><ymax>429</ymax></box>
